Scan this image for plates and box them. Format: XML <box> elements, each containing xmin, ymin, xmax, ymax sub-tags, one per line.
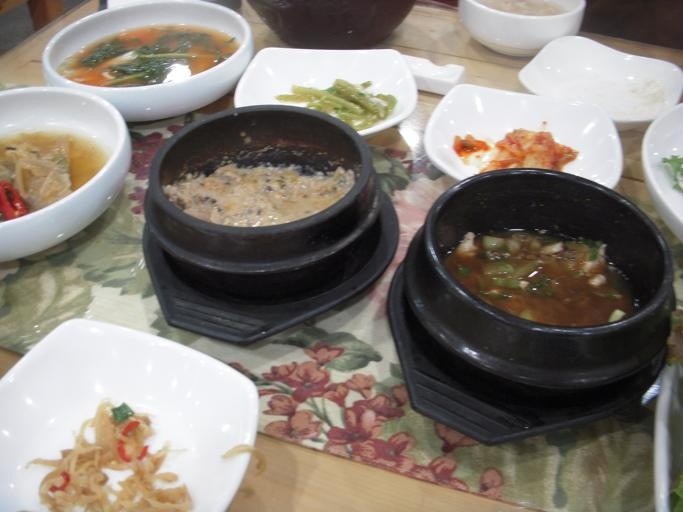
<box><xmin>641</xmin><ymin>104</ymin><xmax>682</xmax><ymax>242</ymax></box>
<box><xmin>421</xmin><ymin>82</ymin><xmax>624</xmax><ymax>191</ymax></box>
<box><xmin>516</xmin><ymin>35</ymin><xmax>683</xmax><ymax>127</ymax></box>
<box><xmin>233</xmin><ymin>45</ymin><xmax>418</xmax><ymax>138</ymax></box>
<box><xmin>0</xmin><ymin>319</ymin><xmax>262</xmax><ymax>511</ymax></box>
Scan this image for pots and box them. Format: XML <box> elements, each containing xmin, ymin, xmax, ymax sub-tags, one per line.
<box><xmin>141</xmin><ymin>105</ymin><xmax>383</xmax><ymax>304</ymax></box>
<box><xmin>400</xmin><ymin>167</ymin><xmax>674</xmax><ymax>413</ymax></box>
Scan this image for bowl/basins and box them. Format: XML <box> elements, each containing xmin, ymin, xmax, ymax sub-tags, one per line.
<box><xmin>248</xmin><ymin>1</ymin><xmax>422</xmax><ymax>50</ymax></box>
<box><xmin>0</xmin><ymin>88</ymin><xmax>132</xmax><ymax>262</ymax></box>
<box><xmin>41</xmin><ymin>0</ymin><xmax>254</xmax><ymax>126</ymax></box>
<box><xmin>461</xmin><ymin>0</ymin><xmax>589</xmax><ymax>61</ymax></box>
